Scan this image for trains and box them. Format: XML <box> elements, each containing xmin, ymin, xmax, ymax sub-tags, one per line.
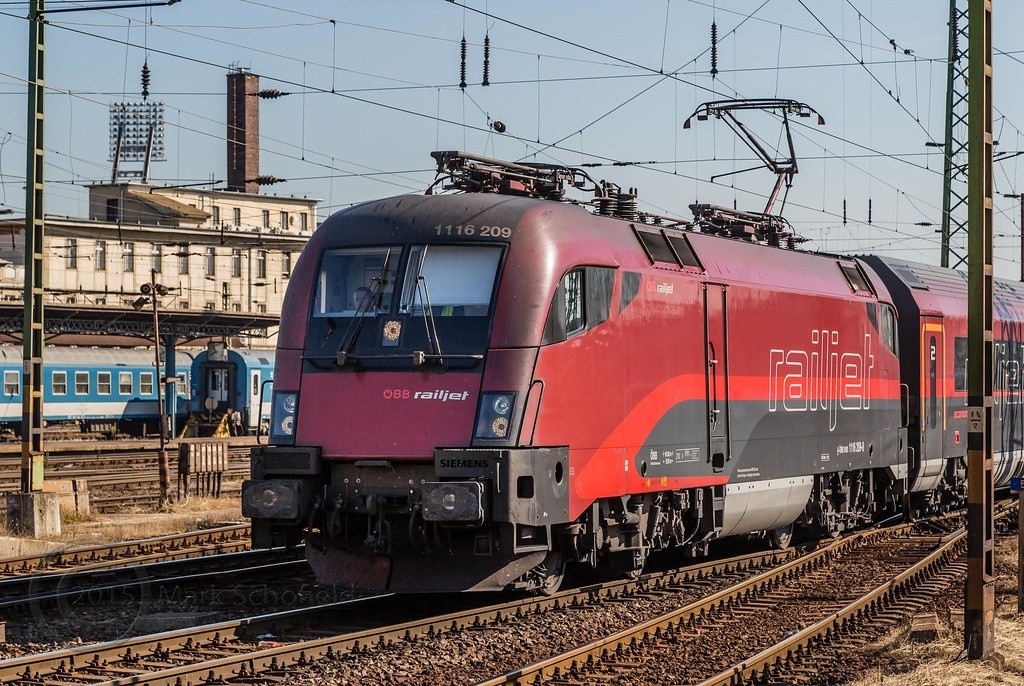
<box><xmin>187</xmin><ymin>348</ymin><xmax>277</xmax><ymax>436</ymax></box>
<box><xmin>0</xmin><ymin>349</ymin><xmax>207</xmax><ymax>436</ymax></box>
<box><xmin>239</xmin><ymin>193</ymin><xmax>1023</xmax><ymax>596</ymax></box>
